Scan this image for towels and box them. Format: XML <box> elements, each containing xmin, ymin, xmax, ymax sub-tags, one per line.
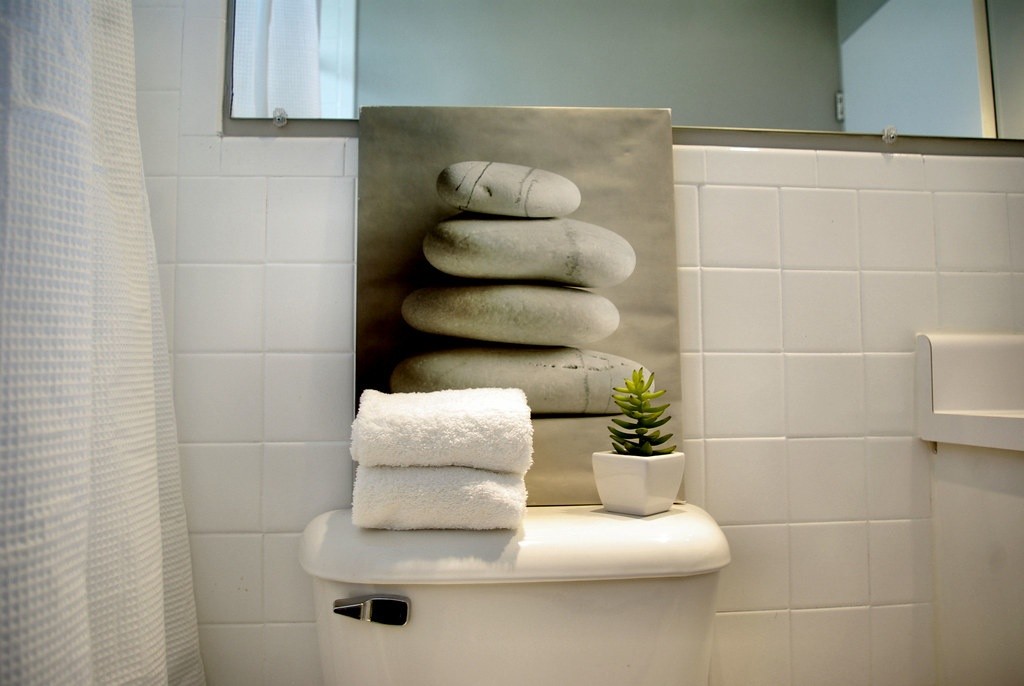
<box><xmin>349</xmin><ymin>386</ymin><xmax>535</xmax><ymax>475</ymax></box>
<box><xmin>351</xmin><ymin>465</ymin><xmax>529</xmax><ymax>531</ymax></box>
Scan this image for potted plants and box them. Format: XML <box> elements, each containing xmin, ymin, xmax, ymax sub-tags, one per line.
<box><xmin>592</xmin><ymin>367</ymin><xmax>687</xmax><ymax>516</ymax></box>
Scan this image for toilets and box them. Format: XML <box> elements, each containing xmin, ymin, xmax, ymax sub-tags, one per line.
<box><xmin>298</xmin><ymin>499</ymin><xmax>732</xmax><ymax>686</ymax></box>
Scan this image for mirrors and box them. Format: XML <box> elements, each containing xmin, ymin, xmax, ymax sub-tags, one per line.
<box><xmin>230</xmin><ymin>0</ymin><xmax>1024</xmax><ymax>143</ymax></box>
